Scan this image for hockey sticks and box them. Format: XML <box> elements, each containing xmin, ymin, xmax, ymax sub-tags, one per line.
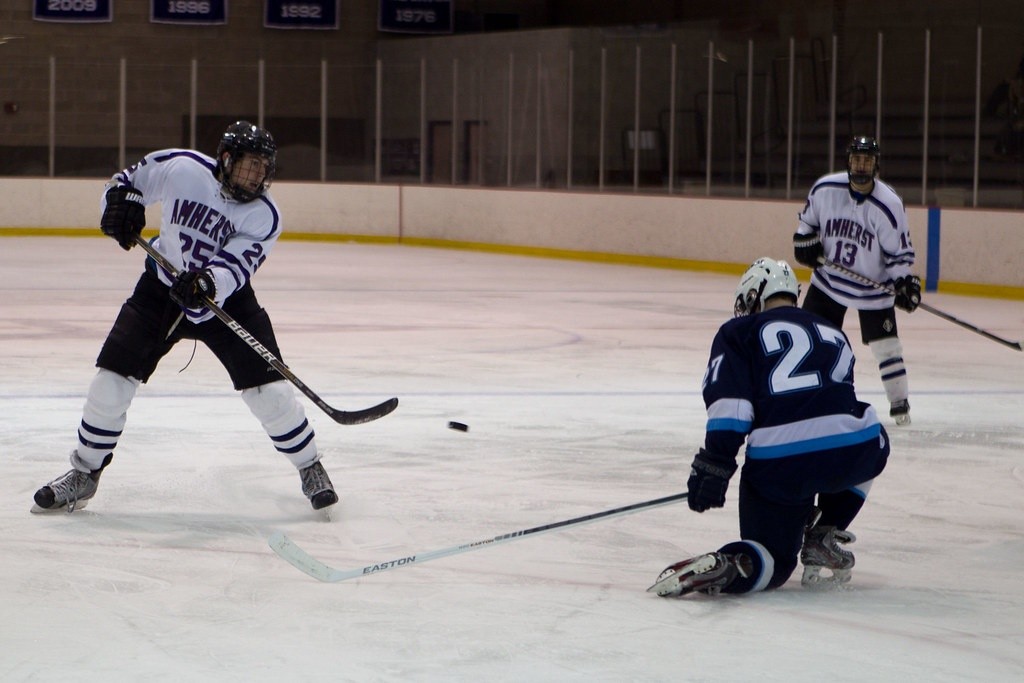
<box><xmin>267</xmin><ymin>492</ymin><xmax>689</xmax><ymax>582</ymax></box>
<box><xmin>135</xmin><ymin>235</ymin><xmax>399</xmax><ymax>426</ymax></box>
<box><xmin>818</xmin><ymin>256</ymin><xmax>1024</xmax><ymax>353</ymax></box>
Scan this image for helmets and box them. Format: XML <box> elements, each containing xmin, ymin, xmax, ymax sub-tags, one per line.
<box><xmin>846</xmin><ymin>136</ymin><xmax>879</xmax><ymax>184</ymax></box>
<box><xmin>216</xmin><ymin>120</ymin><xmax>278</xmax><ymax>203</ymax></box>
<box><xmin>734</xmin><ymin>257</ymin><xmax>802</xmax><ymax>313</ymax></box>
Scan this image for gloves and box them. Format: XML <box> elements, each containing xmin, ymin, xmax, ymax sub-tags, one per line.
<box><xmin>687</xmin><ymin>447</ymin><xmax>738</xmax><ymax>513</ymax></box>
<box><xmin>894</xmin><ymin>275</ymin><xmax>920</xmax><ymax>311</ymax></box>
<box><xmin>793</xmin><ymin>231</ymin><xmax>824</xmax><ymax>268</ymax></box>
<box><xmin>171</xmin><ymin>270</ymin><xmax>215</xmax><ymax>309</ymax></box>
<box><xmin>99</xmin><ymin>184</ymin><xmax>147</xmax><ymax>251</ymax></box>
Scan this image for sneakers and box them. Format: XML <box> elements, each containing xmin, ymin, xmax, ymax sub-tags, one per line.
<box><xmin>29</xmin><ymin>449</ymin><xmax>114</xmax><ymax>514</ymax></box>
<box><xmin>646</xmin><ymin>551</ymin><xmax>752</xmax><ymax>599</ymax></box>
<box><xmin>890</xmin><ymin>398</ymin><xmax>911</xmax><ymax>425</ymax></box>
<box><xmin>801</xmin><ymin>525</ymin><xmax>856</xmax><ymax>588</ymax></box>
<box><xmin>297</xmin><ymin>453</ymin><xmax>339</xmax><ymax>510</ymax></box>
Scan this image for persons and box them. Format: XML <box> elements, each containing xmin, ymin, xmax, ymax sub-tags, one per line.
<box><xmin>31</xmin><ymin>119</ymin><xmax>337</xmax><ymax>525</ymax></box>
<box><xmin>792</xmin><ymin>133</ymin><xmax>922</xmax><ymax>426</ymax></box>
<box><xmin>648</xmin><ymin>256</ymin><xmax>891</xmax><ymax>599</ymax></box>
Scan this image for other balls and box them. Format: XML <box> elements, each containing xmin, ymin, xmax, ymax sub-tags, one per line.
<box><xmin>449</xmin><ymin>421</ymin><xmax>469</xmax><ymax>433</ymax></box>
